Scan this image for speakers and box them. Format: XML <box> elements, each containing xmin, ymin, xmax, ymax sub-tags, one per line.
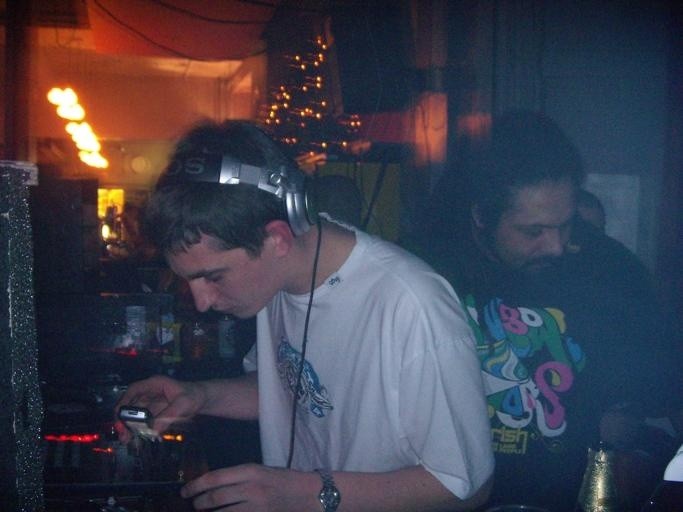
<box><xmin>331</xmin><ymin>0</ymin><xmax>419</xmax><ymax>113</ymax></box>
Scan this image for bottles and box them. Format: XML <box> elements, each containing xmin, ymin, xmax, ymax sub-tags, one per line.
<box><xmin>156</xmin><ymin>294</ymin><xmax>237</xmax><ymax>363</ymax></box>
<box><xmin>575</xmin><ymin>441</ymin><xmax>626</xmax><ymax>512</ymax></box>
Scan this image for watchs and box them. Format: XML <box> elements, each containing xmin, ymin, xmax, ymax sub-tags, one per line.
<box><xmin>313</xmin><ymin>469</ymin><xmax>341</xmax><ymax>512</ymax></box>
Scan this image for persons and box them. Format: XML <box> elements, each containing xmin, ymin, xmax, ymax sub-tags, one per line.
<box><xmin>421</xmin><ymin>107</ymin><xmax>655</xmax><ymax>511</ymax></box>
<box><xmin>113</xmin><ymin>118</ymin><xmax>495</xmax><ymax>512</ymax></box>
<box><xmin>574</xmin><ymin>190</ymin><xmax>605</xmax><ymax>234</ymax></box>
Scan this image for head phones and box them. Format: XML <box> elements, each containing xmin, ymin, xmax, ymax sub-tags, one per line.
<box><xmin>155</xmin><ymin>154</ymin><xmax>319</xmax><ymax>238</ymax></box>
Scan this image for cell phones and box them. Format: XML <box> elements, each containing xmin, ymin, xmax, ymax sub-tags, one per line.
<box><xmin>117</xmin><ymin>406</ymin><xmax>163</xmax><ymax>443</ymax></box>
